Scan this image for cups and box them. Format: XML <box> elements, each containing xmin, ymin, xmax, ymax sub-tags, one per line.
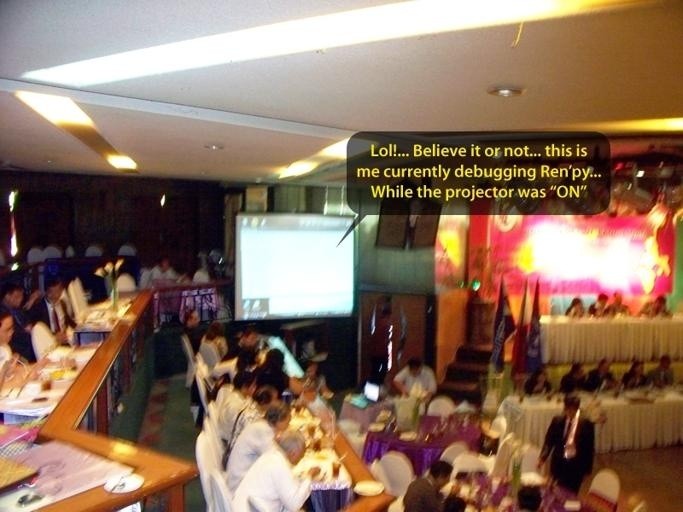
<box><xmin>281</xmin><ymin>391</ymin><xmax>293</xmax><ymax>406</ymax></box>
<box><xmin>42</xmin><ymin>377</ymin><xmax>52</xmax><ymax>391</ymax></box>
<box><xmin>331</xmin><ymin>461</ymin><xmax>343</xmax><ymax>479</ymax></box>
<box><xmin>307</xmin><ymin>423</ymin><xmax>321</xmax><ymax>453</ymax></box>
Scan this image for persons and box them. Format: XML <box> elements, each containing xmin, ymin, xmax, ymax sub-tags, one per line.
<box><xmin>0</xmin><ymin>255</ymin><xmax>234</xmax><ymax>393</ymax></box>
<box><xmin>200</xmin><ymin>292</ymin><xmax>683</xmax><ymax>512</ymax></box>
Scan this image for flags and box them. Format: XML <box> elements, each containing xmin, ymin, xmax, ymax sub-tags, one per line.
<box><xmin>525</xmin><ymin>280</ymin><xmax>545</xmax><ymax>375</ymax></box>
<box><xmin>489</xmin><ymin>278</ymin><xmax>515</xmax><ymax>371</ymax></box>
<box><xmin>510</xmin><ymin>279</ymin><xmax>527</xmax><ymax>389</ymax></box>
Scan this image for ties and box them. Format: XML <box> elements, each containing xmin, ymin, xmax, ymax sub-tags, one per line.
<box><xmin>52</xmin><ymin>308</ymin><xmax>61</xmax><ymax>331</ymax></box>
<box><xmin>564</xmin><ymin>419</ymin><xmax>572</xmax><ymax>446</ymax></box>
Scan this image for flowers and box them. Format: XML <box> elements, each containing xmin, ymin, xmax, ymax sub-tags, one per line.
<box><xmin>95</xmin><ymin>257</ymin><xmax>125</xmax><ymax>289</ymax></box>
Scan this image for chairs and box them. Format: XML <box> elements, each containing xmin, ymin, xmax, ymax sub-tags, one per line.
<box><xmin>179</xmin><ymin>333</ymin><xmax>239</xmax><ymax>512</ymax></box>
<box><xmin>338</xmin><ymin>394</ymin><xmax>623</xmax><ymax>512</ymax></box>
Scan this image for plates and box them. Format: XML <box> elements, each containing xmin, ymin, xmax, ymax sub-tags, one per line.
<box><xmin>353</xmin><ymin>479</ymin><xmax>386</xmax><ymax>497</ymax></box>
<box><xmin>103</xmin><ymin>470</ymin><xmax>145</xmax><ymax>494</ymax></box>
<box><xmin>39</xmin><ymin>368</ymin><xmax>82</xmax><ymax>383</ymax></box>
